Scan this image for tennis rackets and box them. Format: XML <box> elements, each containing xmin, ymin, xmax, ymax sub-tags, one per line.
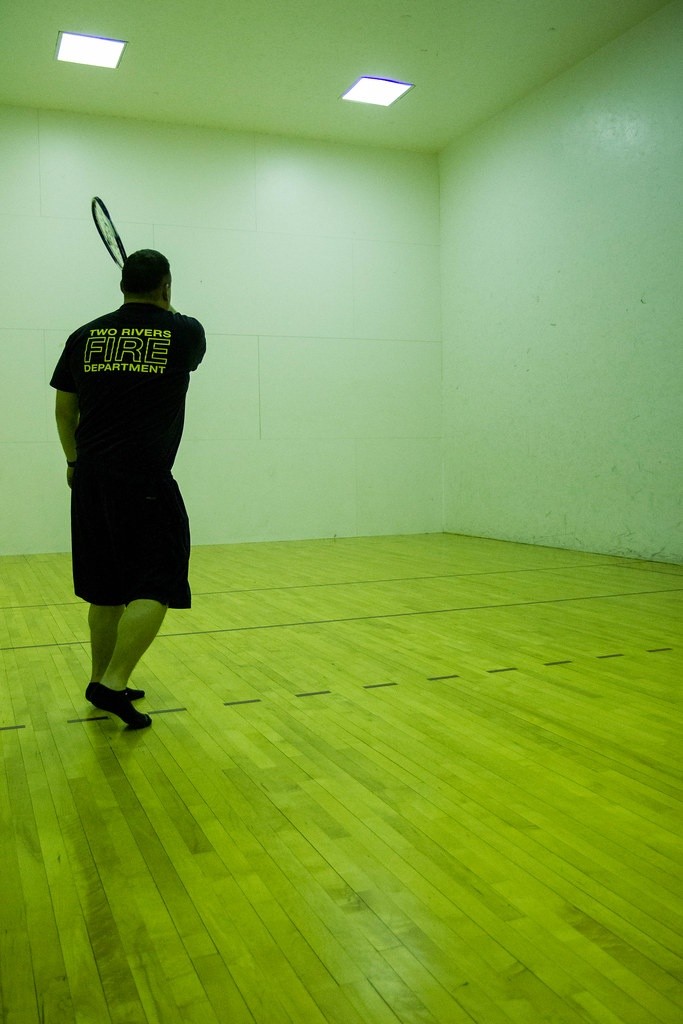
<box><xmin>90</xmin><ymin>196</ymin><xmax>128</xmax><ymax>269</ymax></box>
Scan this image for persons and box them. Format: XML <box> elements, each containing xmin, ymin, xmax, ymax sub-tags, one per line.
<box><xmin>48</xmin><ymin>249</ymin><xmax>207</xmax><ymax>727</ymax></box>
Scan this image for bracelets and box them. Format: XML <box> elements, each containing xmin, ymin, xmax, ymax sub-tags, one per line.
<box><xmin>67</xmin><ymin>460</ymin><xmax>77</xmax><ymax>468</ymax></box>
